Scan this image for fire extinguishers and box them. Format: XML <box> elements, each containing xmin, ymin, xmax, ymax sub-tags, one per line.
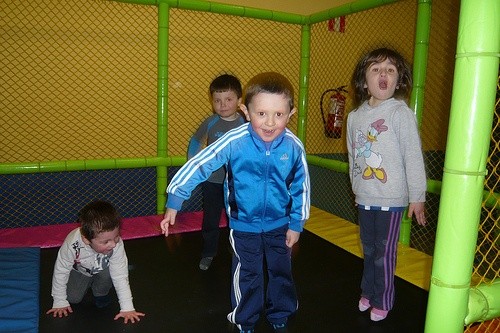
<box><xmin>321</xmin><ymin>85</ymin><xmax>348</xmax><ymax>139</ymax></box>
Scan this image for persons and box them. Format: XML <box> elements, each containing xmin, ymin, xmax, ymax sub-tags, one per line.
<box><xmin>46</xmin><ymin>199</ymin><xmax>145</xmax><ymax>325</ymax></box>
<box><xmin>346</xmin><ymin>46</ymin><xmax>427</xmax><ymax>322</ymax></box>
<box><xmin>188</xmin><ymin>74</ymin><xmax>251</xmax><ymax>271</ymax></box>
<box><xmin>158</xmin><ymin>71</ymin><xmax>312</xmax><ymax>333</ymax></box>
